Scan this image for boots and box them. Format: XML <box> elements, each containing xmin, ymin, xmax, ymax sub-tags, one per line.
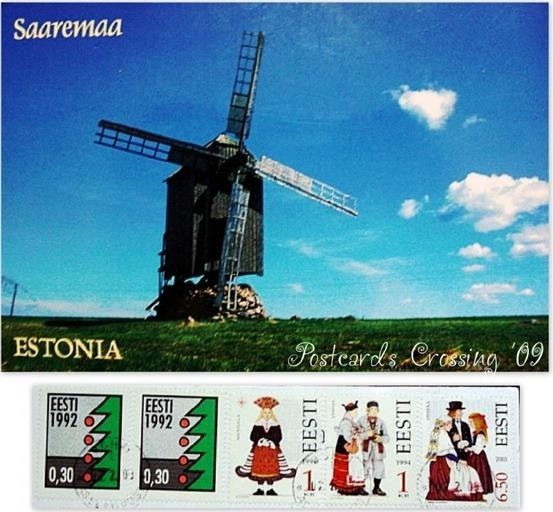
<box><xmin>372</xmin><ymin>479</ymin><xmax>386</xmax><ymax>496</ymax></box>
<box><xmin>360</xmin><ymin>488</ymin><xmax>368</xmax><ymax>495</ymax></box>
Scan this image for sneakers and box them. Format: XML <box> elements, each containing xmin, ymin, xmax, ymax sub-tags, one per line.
<box><xmin>266</xmin><ymin>489</ymin><xmax>277</xmax><ymax>495</ymax></box>
<box><xmin>253</xmin><ymin>488</ymin><xmax>264</xmax><ymax>495</ymax></box>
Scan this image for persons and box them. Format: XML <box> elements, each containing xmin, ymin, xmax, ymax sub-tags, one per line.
<box><xmin>329</xmin><ymin>401</ymin><xmax>390</xmax><ymax>495</ymax></box>
<box><xmin>236</xmin><ymin>397</ymin><xmax>296</xmax><ymax>495</ymax></box>
<box><xmin>425</xmin><ymin>401</ymin><xmax>492</xmax><ymax>501</ymax></box>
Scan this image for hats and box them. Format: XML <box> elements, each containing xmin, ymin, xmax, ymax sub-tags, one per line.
<box><xmin>254</xmin><ymin>397</ymin><xmax>279</xmax><ymax>408</ymax></box>
<box><xmin>445</xmin><ymin>401</ymin><xmax>465</xmax><ymax>410</ymax></box>
<box><xmin>367</xmin><ymin>402</ymin><xmax>378</xmax><ymax>407</ymax></box>
<box><xmin>342</xmin><ymin>400</ymin><xmax>358</xmax><ymax>411</ymax></box>
